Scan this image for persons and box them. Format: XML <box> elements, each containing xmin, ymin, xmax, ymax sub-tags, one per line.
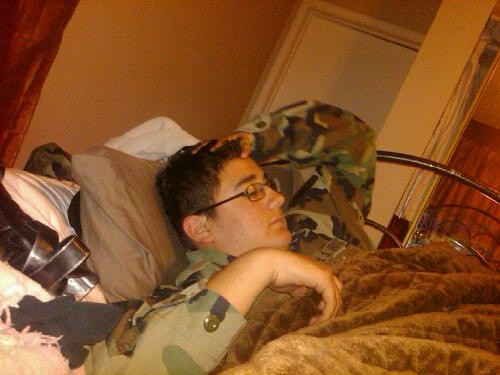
<box><xmin>84</xmin><ymin>98</ymin><xmax>500</xmax><ymax>375</ymax></box>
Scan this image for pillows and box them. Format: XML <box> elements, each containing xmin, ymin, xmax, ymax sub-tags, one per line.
<box><xmin>69</xmin><ymin>146</ymin><xmax>189</xmax><ymax>303</ymax></box>
<box><xmin>3</xmin><ymin>115</ymin><xmax>202</xmax><ymax>297</ymax></box>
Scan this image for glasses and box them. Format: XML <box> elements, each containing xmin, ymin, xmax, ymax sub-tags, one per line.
<box><xmin>180</xmin><ymin>177</ymin><xmax>282</xmax><ymax>233</ymax></box>
